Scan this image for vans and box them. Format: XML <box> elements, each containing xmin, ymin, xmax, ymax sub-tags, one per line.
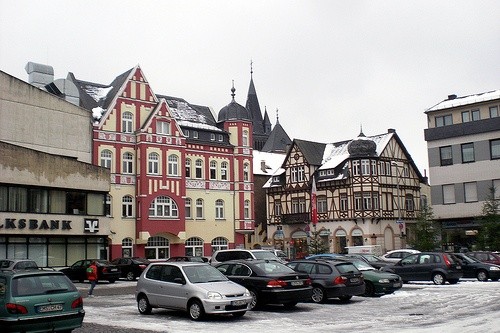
<box><xmin>207</xmin><ymin>249</ymin><xmax>286</xmax><ymax>272</ymax></box>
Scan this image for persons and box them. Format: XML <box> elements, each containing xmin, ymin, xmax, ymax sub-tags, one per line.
<box><xmin>87</xmin><ymin>260</ymin><xmax>97</xmax><ymax>298</ymax></box>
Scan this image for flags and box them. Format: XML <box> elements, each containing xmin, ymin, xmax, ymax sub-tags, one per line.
<box><xmin>311</xmin><ymin>180</ymin><xmax>318</xmax><ymax>224</ymax></box>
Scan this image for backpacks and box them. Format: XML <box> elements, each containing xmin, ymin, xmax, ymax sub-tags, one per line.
<box><xmin>86</xmin><ymin>268</ymin><xmax>93</xmax><ymax>276</ymax></box>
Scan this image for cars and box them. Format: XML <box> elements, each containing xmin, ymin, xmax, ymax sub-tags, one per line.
<box><xmin>447</xmin><ymin>253</ymin><xmax>500</xmax><ymax>282</ymax></box>
<box><xmin>207</xmin><ymin>259</ymin><xmax>314</xmax><ymax>310</ymax></box>
<box><xmin>350</xmin><ymin>252</ymin><xmax>396</xmax><ymax>270</ymax></box>
<box><xmin>380</xmin><ymin>248</ymin><xmax>430</xmax><ymax>265</ymax></box>
<box><xmin>378</xmin><ymin>252</ymin><xmax>464</xmax><ymax>285</ymax></box>
<box><xmin>314</xmin><ymin>257</ymin><xmax>403</xmax><ymax>298</ymax></box>
<box><xmin>135</xmin><ymin>261</ymin><xmax>253</xmax><ymax>321</ymax></box>
<box><xmin>110</xmin><ymin>257</ymin><xmax>151</xmax><ymax>281</ymax></box>
<box><xmin>284</xmin><ymin>259</ymin><xmax>367</xmax><ymax>303</ymax></box>
<box><xmin>163</xmin><ymin>256</ymin><xmax>209</xmax><ymax>262</ymax></box>
<box><xmin>466</xmin><ymin>251</ymin><xmax>500</xmax><ymax>265</ymax></box>
<box><xmin>58</xmin><ymin>259</ymin><xmax>122</xmax><ymax>284</ymax></box>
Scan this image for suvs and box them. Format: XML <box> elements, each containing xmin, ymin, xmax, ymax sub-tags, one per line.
<box><xmin>0</xmin><ymin>260</ymin><xmax>39</xmax><ymax>273</ymax></box>
<box><xmin>0</xmin><ymin>267</ymin><xmax>86</xmax><ymax>333</ymax></box>
